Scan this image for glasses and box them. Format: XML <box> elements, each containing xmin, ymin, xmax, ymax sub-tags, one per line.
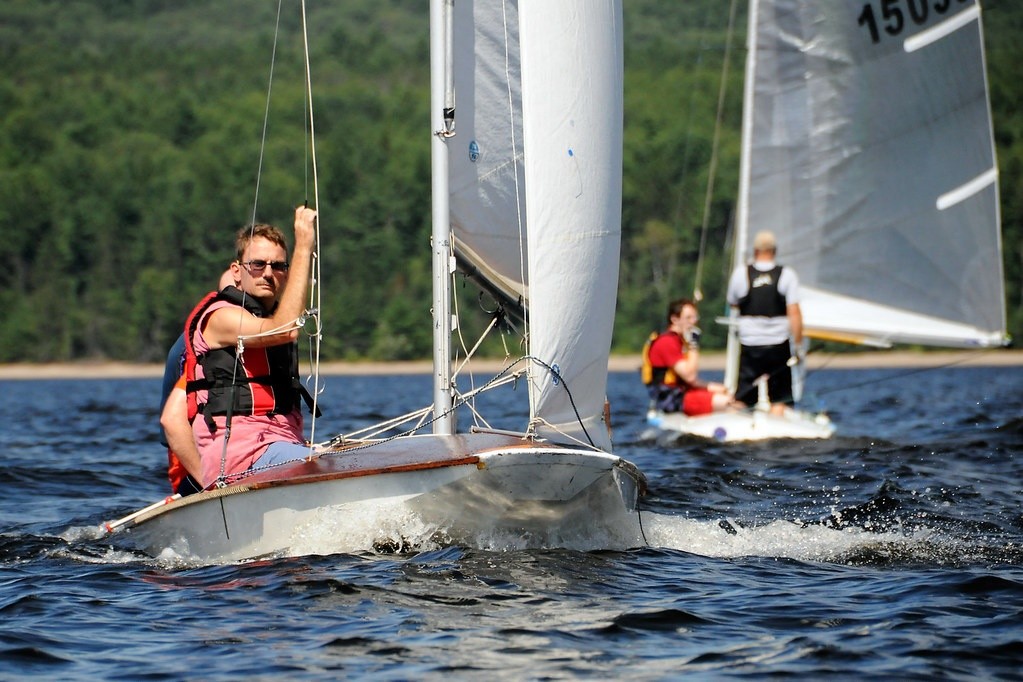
<box><xmin>238</xmin><ymin>259</ymin><xmax>288</xmax><ymax>271</ymax></box>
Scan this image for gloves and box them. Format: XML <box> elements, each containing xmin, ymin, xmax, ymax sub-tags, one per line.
<box><xmin>682</xmin><ymin>327</ymin><xmax>702</xmax><ymax>348</ymax></box>
<box><xmin>795</xmin><ymin>346</ymin><xmax>804</xmax><ymax>364</ymax></box>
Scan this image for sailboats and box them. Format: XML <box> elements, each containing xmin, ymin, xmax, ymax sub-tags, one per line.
<box><xmin>95</xmin><ymin>0</ymin><xmax>648</xmax><ymax>563</ymax></box>
<box><xmin>635</xmin><ymin>0</ymin><xmax>1013</xmax><ymax>442</ymax></box>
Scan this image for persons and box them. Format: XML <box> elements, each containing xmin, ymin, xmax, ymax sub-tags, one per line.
<box><xmin>184</xmin><ymin>206</ymin><xmax>319</xmax><ymax>491</ymax></box>
<box><xmin>159</xmin><ymin>268</ymin><xmax>238</xmax><ymax>449</ymax></box>
<box><xmin>641</xmin><ymin>301</ymin><xmax>745</xmax><ymax>417</ymax></box>
<box><xmin>160</xmin><ymin>362</ymin><xmax>203</xmax><ymax>499</ymax></box>
<box><xmin>726</xmin><ymin>230</ymin><xmax>805</xmax><ymax>414</ymax></box>
